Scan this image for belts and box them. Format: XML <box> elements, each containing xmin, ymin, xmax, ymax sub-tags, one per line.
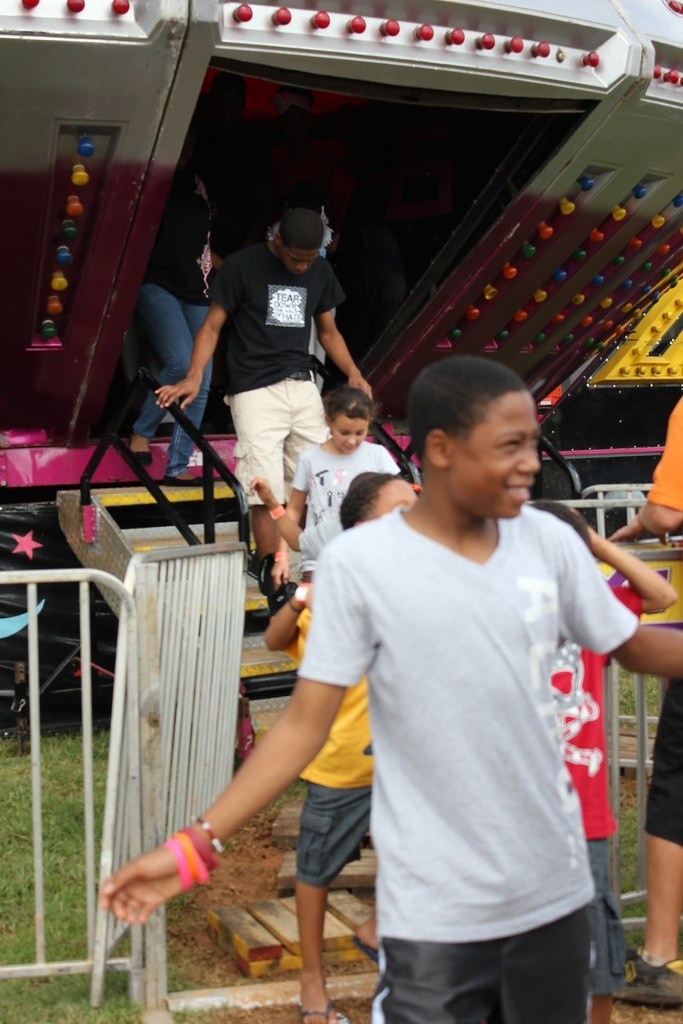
<box><xmin>287</xmin><ymin>372</ymin><xmax>314</xmax><ymax>380</ymax></box>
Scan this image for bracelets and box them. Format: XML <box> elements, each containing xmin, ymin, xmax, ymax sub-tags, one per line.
<box><xmin>163</xmin><ymin>817</ymin><xmax>225</xmax><ymax>888</ymax></box>
<box><xmin>288</xmin><ymin>600</ymin><xmax>304</xmax><ymax>614</ymax></box>
<box><xmin>294</xmin><ymin>583</ymin><xmax>311</xmax><ymax>609</ymax></box>
<box><xmin>269</xmin><ymin>505</ymin><xmax>287</xmax><ymax>520</ymax></box>
<box><xmin>274</xmin><ymin>550</ymin><xmax>290</xmax><ymax>563</ymax></box>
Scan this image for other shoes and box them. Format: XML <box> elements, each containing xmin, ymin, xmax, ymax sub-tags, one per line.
<box><xmin>248</xmin><ymin>550</ymin><xmax>263</xmax><ymax>579</ymax></box>
<box><xmin>164</xmin><ymin>474</ymin><xmax>206</xmax><ymax>487</ymax></box>
<box><xmin>129</xmin><ymin>434</ymin><xmax>152</xmax><ymax>465</ymax></box>
<box><xmin>259</xmin><ymin>552</ymin><xmax>278</xmax><ymax>595</ymax></box>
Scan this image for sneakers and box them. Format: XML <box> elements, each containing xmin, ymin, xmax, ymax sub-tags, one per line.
<box><xmin>612</xmin><ymin>945</ymin><xmax>683</xmax><ymax>1003</ymax></box>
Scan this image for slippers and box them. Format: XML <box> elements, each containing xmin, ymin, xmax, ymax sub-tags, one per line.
<box><xmin>301</xmin><ymin>1002</ymin><xmax>338</xmax><ymax>1024</ymax></box>
<box><xmin>352</xmin><ymin>938</ymin><xmax>379</xmax><ymax>961</ymax></box>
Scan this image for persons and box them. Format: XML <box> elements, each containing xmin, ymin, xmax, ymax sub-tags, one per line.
<box><xmin>263</xmin><ymin>471</ymin><xmax>418</xmax><ymax>1024</ymax></box>
<box><xmin>605</xmin><ymin>393</ymin><xmax>683</xmax><ymax>1008</ymax></box>
<box><xmin>128</xmin><ymin>131</ymin><xmax>223</xmax><ymax>488</ymax></box>
<box><xmin>272</xmin><ymin>386</ymin><xmax>405</xmax><ymax>592</ymax></box>
<box><xmin>154</xmin><ymin>208</ymin><xmax>372</xmax><ymax>596</ymax></box>
<box><xmin>91</xmin><ymin>72</ymin><xmax>407</xmax><ymax>437</ymax></box>
<box><xmin>528</xmin><ymin>501</ymin><xmax>677</xmax><ymax>1024</ymax></box>
<box><xmin>100</xmin><ymin>358</ymin><xmax>683</xmax><ymax>1024</ymax></box>
